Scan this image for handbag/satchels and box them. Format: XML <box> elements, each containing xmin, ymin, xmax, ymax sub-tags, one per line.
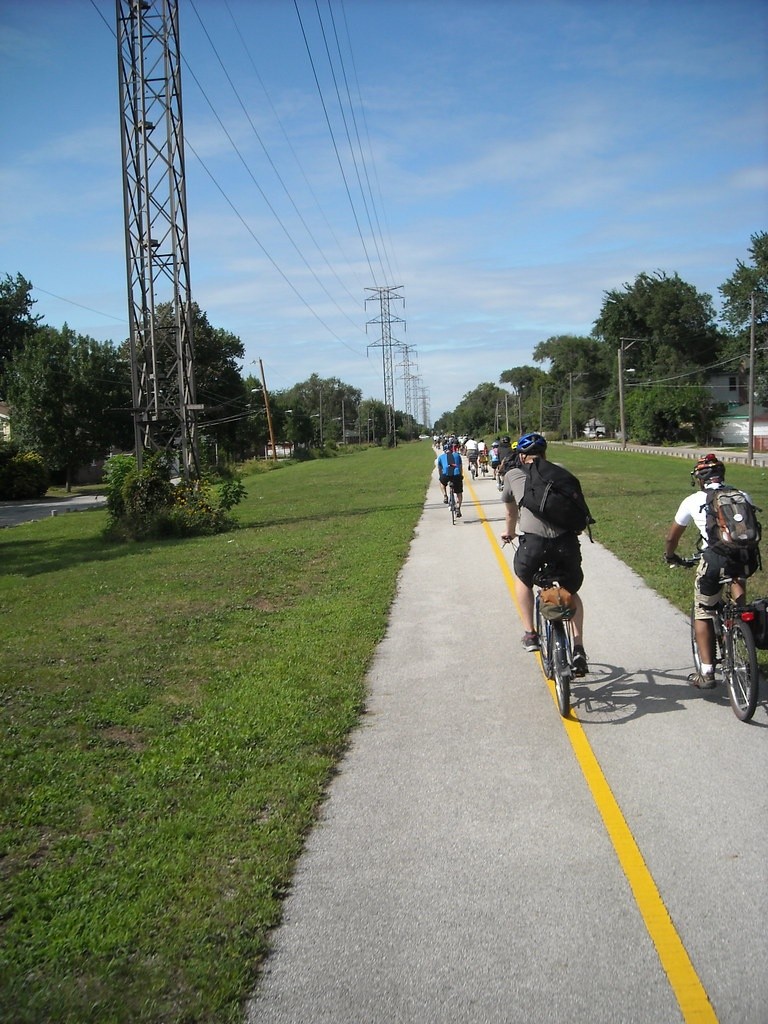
<box><xmin>750</xmin><ymin>597</ymin><xmax>768</xmax><ymax>650</ymax></box>
<box><xmin>539</xmin><ymin>586</ymin><xmax>577</xmax><ymax>622</ymax></box>
<box><xmin>479</xmin><ymin>456</ymin><xmax>488</xmax><ymax>462</ymax></box>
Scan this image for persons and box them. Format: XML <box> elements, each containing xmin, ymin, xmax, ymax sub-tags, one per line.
<box><xmin>501</xmin><ymin>432</ymin><xmax>590</xmax><ymax>674</ymax></box>
<box><xmin>431</xmin><ymin>429</ymin><xmax>518</xmax><ymax>489</ymax></box>
<box><xmin>437</xmin><ymin>444</ymin><xmax>464</xmax><ymax>517</ymax></box>
<box><xmin>666</xmin><ymin>454</ymin><xmax>762</xmax><ymax>689</ymax></box>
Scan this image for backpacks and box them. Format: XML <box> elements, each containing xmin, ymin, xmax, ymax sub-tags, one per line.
<box><xmin>507</xmin><ymin>451</ymin><xmax>521</xmax><ymax>470</ymax></box>
<box><xmin>516</xmin><ymin>457</ymin><xmax>596</xmax><ymax>531</ymax></box>
<box><xmin>699</xmin><ymin>486</ymin><xmax>762</xmax><ymax>558</ymax></box>
<box><xmin>493</xmin><ymin>448</ymin><xmax>501</xmax><ymax>455</ymax></box>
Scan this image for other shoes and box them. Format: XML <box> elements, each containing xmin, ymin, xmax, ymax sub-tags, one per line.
<box><xmin>443</xmin><ymin>496</ymin><xmax>448</xmax><ymax>503</ymax></box>
<box><xmin>731</xmin><ymin>620</ymin><xmax>743</xmax><ymax>639</ymax></box>
<box><xmin>687</xmin><ymin>669</ymin><xmax>718</xmax><ymax>689</ymax></box>
<box><xmin>521</xmin><ymin>632</ymin><xmax>539</xmax><ymax>651</ymax></box>
<box><xmin>454</xmin><ymin>508</ymin><xmax>462</xmax><ymax>517</ymax></box>
<box><xmin>572</xmin><ymin>650</ymin><xmax>590</xmax><ymax>674</ymax></box>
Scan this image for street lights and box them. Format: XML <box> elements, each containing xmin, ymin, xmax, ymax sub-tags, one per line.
<box><xmin>251</xmin><ymin>388</ymin><xmax>278</xmax><ymax>463</ymax></box>
<box><xmin>618</xmin><ymin>348</ymin><xmax>636</xmax><ymax>449</ymax></box>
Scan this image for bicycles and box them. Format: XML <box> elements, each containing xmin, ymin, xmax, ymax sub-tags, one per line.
<box><xmin>439</xmin><ymin>474</ymin><xmax>464</xmax><ymax>525</ymax></box>
<box><xmin>499</xmin><ymin>530</ymin><xmax>586</xmax><ymax>718</ymax></box>
<box><xmin>432</xmin><ymin>442</ymin><xmax>500</xmax><ymax>480</ymax></box>
<box><xmin>665</xmin><ymin>547</ymin><xmax>759</xmax><ymax>722</ymax></box>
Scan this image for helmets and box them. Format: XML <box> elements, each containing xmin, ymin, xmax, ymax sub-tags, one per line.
<box><xmin>491</xmin><ymin>442</ymin><xmax>499</xmax><ymax>447</ymax></box>
<box><xmin>443</xmin><ymin>444</ymin><xmax>454</xmax><ymax>451</ymax></box>
<box><xmin>694</xmin><ymin>454</ymin><xmax>726</xmax><ymax>490</ymax></box>
<box><xmin>518</xmin><ymin>433</ymin><xmax>547</xmax><ymax>453</ymax></box>
<box><xmin>502</xmin><ymin>437</ymin><xmax>510</xmax><ymax>443</ymax></box>
<box><xmin>435</xmin><ymin>432</ymin><xmax>501</xmax><ymax>442</ymax></box>
<box><xmin>511</xmin><ymin>441</ymin><xmax>518</xmax><ymax>453</ymax></box>
